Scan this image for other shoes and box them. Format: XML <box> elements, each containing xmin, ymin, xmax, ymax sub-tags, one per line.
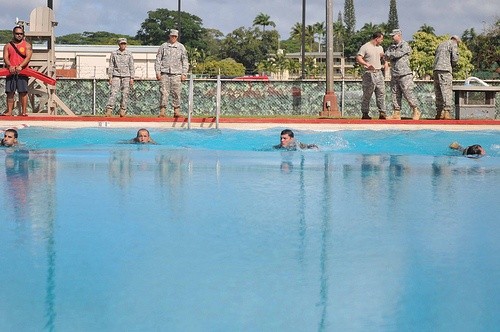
<box><xmin>362</xmin><ymin>114</ymin><xmax>371</xmax><ymax>120</ymax></box>
<box><xmin>412</xmin><ymin>107</ymin><xmax>421</xmax><ymax>120</ymax></box>
<box><xmin>378</xmin><ymin>112</ymin><xmax>387</xmax><ymax>119</ymax></box>
<box><xmin>436</xmin><ymin>114</ymin><xmax>444</xmax><ymax>119</ymax></box>
<box><xmin>444</xmin><ymin>112</ymin><xmax>455</xmax><ymax>120</ymax></box>
<box><xmin>21</xmin><ymin>113</ymin><xmax>27</xmax><ymax>116</ymax></box>
<box><xmin>386</xmin><ymin>111</ymin><xmax>401</xmax><ymax>120</ymax></box>
<box><xmin>106</xmin><ymin>110</ymin><xmax>111</xmax><ymax>117</ymax></box>
<box><xmin>158</xmin><ymin>112</ymin><xmax>166</xmax><ymax>118</ymax></box>
<box><xmin>119</xmin><ymin>109</ymin><xmax>125</xmax><ymax>116</ymax></box>
<box><xmin>4</xmin><ymin>113</ymin><xmax>14</xmax><ymax>116</ymax></box>
<box><xmin>173</xmin><ymin>111</ymin><xmax>183</xmax><ymax>119</ymax></box>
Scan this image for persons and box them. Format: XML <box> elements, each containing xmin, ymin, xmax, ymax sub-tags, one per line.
<box><xmin>102</xmin><ymin>38</ymin><xmax>135</xmax><ymax>117</ymax></box>
<box><xmin>380</xmin><ymin>30</ymin><xmax>421</xmax><ymax>120</ymax></box>
<box><xmin>433</xmin><ymin>35</ymin><xmax>460</xmax><ymax>120</ymax></box>
<box><xmin>356</xmin><ymin>31</ymin><xmax>389</xmax><ymax>120</ymax></box>
<box><xmin>154</xmin><ymin>28</ymin><xmax>189</xmax><ymax>118</ymax></box>
<box><xmin>130</xmin><ymin>129</ymin><xmax>155</xmax><ymax>144</ymax></box>
<box><xmin>2</xmin><ymin>27</ymin><xmax>33</xmax><ymax>116</ymax></box>
<box><xmin>269</xmin><ymin>129</ymin><xmax>318</xmax><ymax>151</ymax></box>
<box><xmin>448</xmin><ymin>142</ymin><xmax>484</xmax><ymax>158</ymax></box>
<box><xmin>0</xmin><ymin>129</ymin><xmax>19</xmax><ymax>148</ymax></box>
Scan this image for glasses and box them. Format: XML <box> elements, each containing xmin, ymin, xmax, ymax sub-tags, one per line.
<box><xmin>15</xmin><ymin>32</ymin><xmax>24</xmax><ymax>35</ymax></box>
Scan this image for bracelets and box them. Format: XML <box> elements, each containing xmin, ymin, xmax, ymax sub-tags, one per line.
<box><xmin>19</xmin><ymin>64</ymin><xmax>23</xmax><ymax>69</ymax></box>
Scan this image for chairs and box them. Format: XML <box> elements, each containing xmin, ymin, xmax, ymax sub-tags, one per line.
<box><xmin>17</xmin><ymin>7</ymin><xmax>58</xmax><ymax>49</ymax></box>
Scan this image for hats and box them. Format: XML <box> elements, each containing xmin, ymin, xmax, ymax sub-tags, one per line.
<box><xmin>389</xmin><ymin>29</ymin><xmax>402</xmax><ymax>35</ymax></box>
<box><xmin>118</xmin><ymin>38</ymin><xmax>128</xmax><ymax>44</ymax></box>
<box><xmin>169</xmin><ymin>30</ymin><xmax>179</xmax><ymax>37</ymax></box>
<box><xmin>451</xmin><ymin>34</ymin><xmax>461</xmax><ymax>47</ymax></box>
